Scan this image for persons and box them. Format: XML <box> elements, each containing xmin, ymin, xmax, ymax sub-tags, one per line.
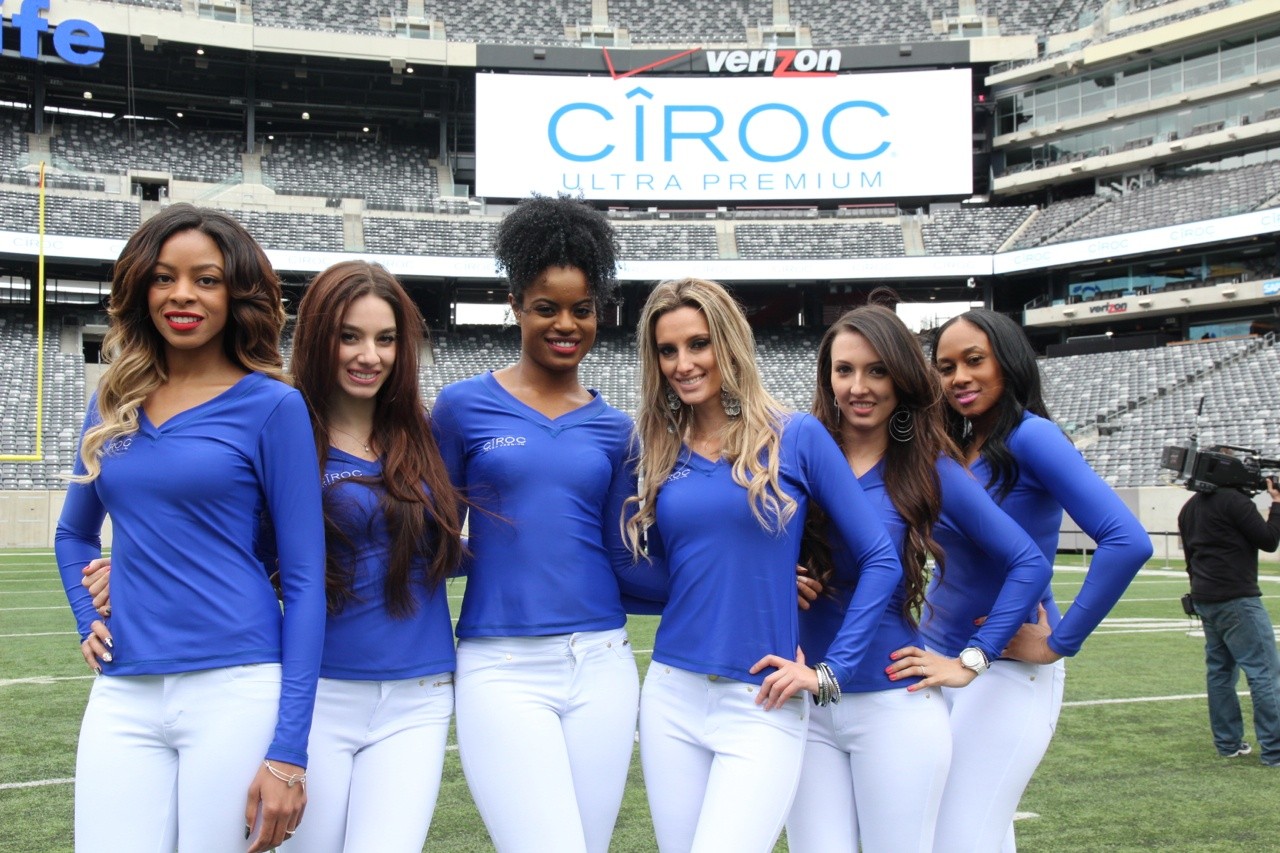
<box><xmin>920</xmin><ymin>311</ymin><xmax>1153</xmax><ymax>853</ymax></box>
<box><xmin>427</xmin><ymin>193</ymin><xmax>823</xmax><ymax>853</ymax></box>
<box><xmin>1177</xmin><ymin>447</ymin><xmax>1280</xmax><ymax>764</ymax></box>
<box><xmin>82</xmin><ymin>260</ymin><xmax>467</xmax><ymax>853</ymax></box>
<box><xmin>619</xmin><ymin>277</ymin><xmax>904</xmax><ymax>853</ymax></box>
<box><xmin>785</xmin><ymin>304</ymin><xmax>1053</xmax><ymax>853</ymax></box>
<box><xmin>55</xmin><ymin>202</ymin><xmax>328</xmax><ymax>853</ymax></box>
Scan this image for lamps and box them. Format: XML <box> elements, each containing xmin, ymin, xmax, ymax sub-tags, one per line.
<box><xmin>899</xmin><ymin>45</ymin><xmax>912</xmax><ymax>55</ymax></box>
<box><xmin>534</xmin><ymin>48</ymin><xmax>546</xmax><ymax>59</ymax></box>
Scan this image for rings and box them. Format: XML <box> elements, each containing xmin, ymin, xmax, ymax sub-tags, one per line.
<box><xmin>286</xmin><ymin>831</ymin><xmax>296</xmax><ymax>834</ymax></box>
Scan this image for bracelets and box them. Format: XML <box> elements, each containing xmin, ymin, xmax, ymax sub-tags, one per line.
<box><xmin>812</xmin><ymin>663</ymin><xmax>842</xmax><ymax>708</ymax></box>
<box><xmin>264</xmin><ymin>760</ymin><xmax>306</xmax><ymax>787</ymax></box>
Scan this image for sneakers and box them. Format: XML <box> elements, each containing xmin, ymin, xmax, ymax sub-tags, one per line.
<box><xmin>1227</xmin><ymin>741</ymin><xmax>1251</xmax><ymax>758</ymax></box>
<box><xmin>1263</xmin><ymin>761</ymin><xmax>1280</xmax><ymax>767</ymax></box>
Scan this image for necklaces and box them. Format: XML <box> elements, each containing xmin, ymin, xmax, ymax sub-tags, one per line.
<box><xmin>328</xmin><ymin>426</ymin><xmax>369</xmax><ymax>452</ymax></box>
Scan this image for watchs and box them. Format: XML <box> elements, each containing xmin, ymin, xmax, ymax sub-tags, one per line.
<box><xmin>960</xmin><ymin>648</ymin><xmax>987</xmax><ymax>674</ymax></box>
